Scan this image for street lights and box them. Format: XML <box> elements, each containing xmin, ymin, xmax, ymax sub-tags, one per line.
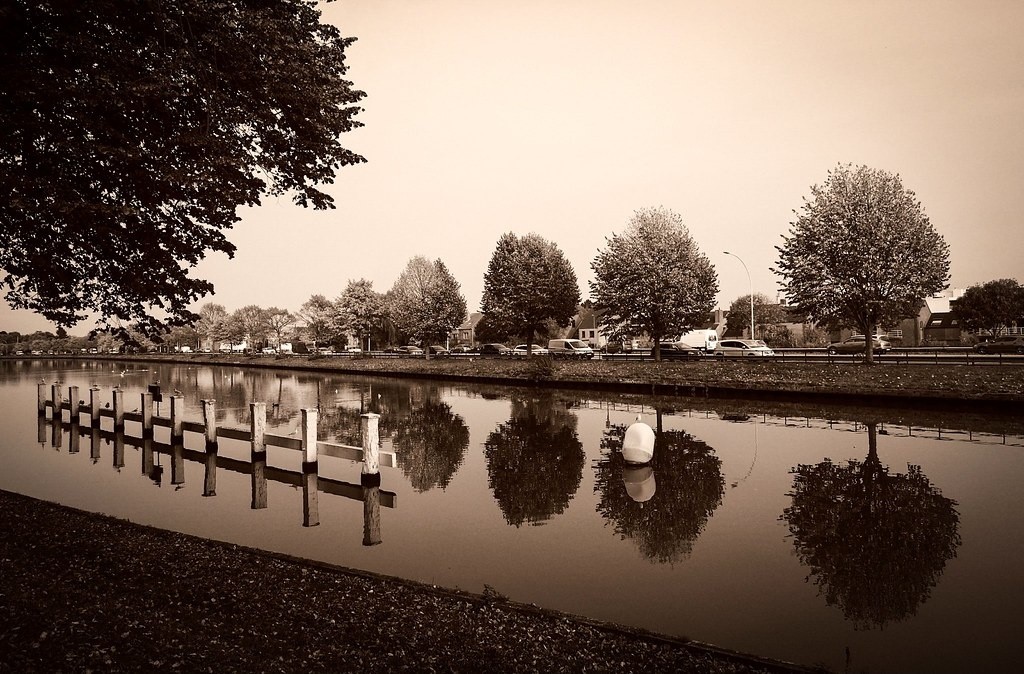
<box><xmin>724</xmin><ymin>251</ymin><xmax>755</xmax><ymax>341</ymax></box>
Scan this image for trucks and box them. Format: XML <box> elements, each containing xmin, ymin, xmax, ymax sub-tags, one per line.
<box><xmin>16</xmin><ymin>351</ymin><xmax>24</xmax><ymax>356</ymax></box>
<box><xmin>202</xmin><ymin>348</ymin><xmax>210</xmax><ymax>353</ymax></box>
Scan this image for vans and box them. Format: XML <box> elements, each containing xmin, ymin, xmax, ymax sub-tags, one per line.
<box><xmin>549</xmin><ymin>339</ymin><xmax>594</xmax><ymax>359</ymax></box>
<box><xmin>81</xmin><ymin>349</ymin><xmax>87</xmax><ymax>354</ymax></box>
<box><xmin>90</xmin><ymin>348</ymin><xmax>97</xmax><ymax>355</ymax></box>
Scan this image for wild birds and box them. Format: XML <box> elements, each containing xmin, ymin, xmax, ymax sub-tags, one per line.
<box><xmin>35</xmin><ymin>374</ymin><xmax>281</xmax><ymax>412</ymax></box>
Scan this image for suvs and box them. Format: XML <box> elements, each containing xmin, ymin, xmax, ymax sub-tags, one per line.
<box><xmin>318</xmin><ymin>348</ymin><xmax>332</xmax><ymax>355</ymax></box>
<box><xmin>479</xmin><ymin>343</ymin><xmax>512</xmax><ymax>357</ymax></box>
<box><xmin>399</xmin><ymin>346</ymin><xmax>423</xmax><ymax>358</ymax></box>
<box><xmin>348</xmin><ymin>346</ymin><xmax>361</xmax><ymax>353</ymax></box>
<box><xmin>600</xmin><ymin>340</ymin><xmax>633</xmax><ymax>354</ymax></box>
<box><xmin>452</xmin><ymin>344</ymin><xmax>471</xmax><ymax>353</ymax></box>
<box><xmin>973</xmin><ymin>335</ymin><xmax>1024</xmax><ymax>355</ymax></box>
<box><xmin>650</xmin><ymin>342</ymin><xmax>703</xmax><ymax>360</ymax></box>
<box><xmin>713</xmin><ymin>340</ymin><xmax>774</xmax><ymax>362</ymax></box>
<box><xmin>827</xmin><ymin>335</ymin><xmax>892</xmax><ymax>356</ymax></box>
<box><xmin>512</xmin><ymin>345</ymin><xmax>548</xmax><ymax>359</ymax></box>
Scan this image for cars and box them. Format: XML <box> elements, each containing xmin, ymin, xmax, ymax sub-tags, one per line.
<box><xmin>423</xmin><ymin>345</ymin><xmax>451</xmax><ymax>357</ymax></box>
<box><xmin>383</xmin><ymin>347</ymin><xmax>399</xmax><ymax>353</ymax></box>
<box><xmin>243</xmin><ymin>348</ymin><xmax>256</xmax><ymax>355</ymax></box>
<box><xmin>262</xmin><ymin>347</ymin><xmax>276</xmax><ymax>355</ymax></box>
